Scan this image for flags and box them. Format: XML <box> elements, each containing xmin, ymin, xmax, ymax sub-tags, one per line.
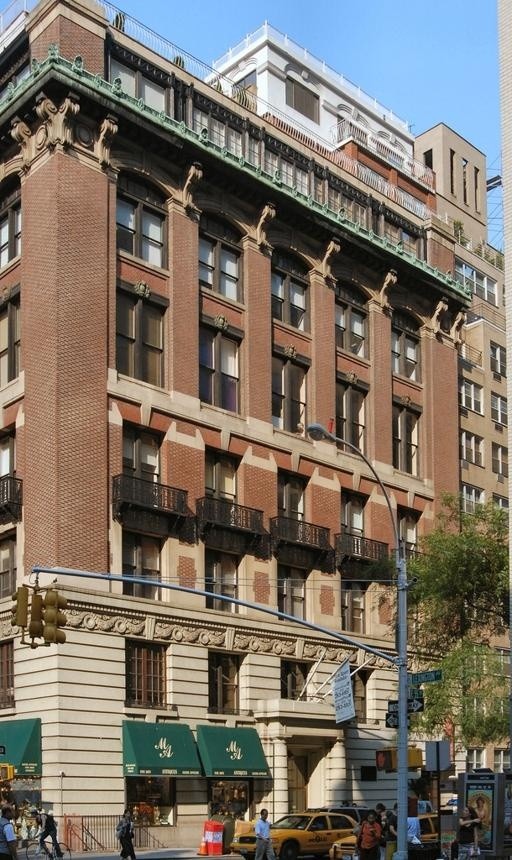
<box><xmin>331</xmin><ymin>659</ymin><xmax>356</xmax><ymax>724</ymax></box>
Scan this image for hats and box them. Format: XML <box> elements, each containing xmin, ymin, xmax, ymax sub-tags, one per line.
<box><xmin>29</xmin><ymin>809</ymin><xmax>37</xmax><ymax>812</ymax></box>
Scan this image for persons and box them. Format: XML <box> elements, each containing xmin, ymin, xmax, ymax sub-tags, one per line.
<box><xmin>473</xmin><ymin>796</ymin><xmax>487</xmax><ymax>823</ymax></box>
<box><xmin>255</xmin><ymin>809</ymin><xmax>277</xmax><ymax>860</ymax></box>
<box><xmin>116</xmin><ymin>809</ymin><xmax>138</xmax><ymax>860</ymax></box>
<box><xmin>458</xmin><ymin>807</ymin><xmax>481</xmax><ymax>859</ymax></box>
<box><xmin>340</xmin><ymin>801</ymin><xmax>420</xmax><ymax>860</ymax></box>
<box><xmin>31</xmin><ymin>809</ymin><xmax>64</xmax><ymax>860</ymax></box>
<box><xmin>1</xmin><ymin>804</ymin><xmax>19</xmax><ymax>860</ymax></box>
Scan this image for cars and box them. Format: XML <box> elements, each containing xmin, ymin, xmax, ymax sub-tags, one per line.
<box><xmin>228</xmin><ymin>797</ymin><xmax>458</xmax><ymax>860</ymax></box>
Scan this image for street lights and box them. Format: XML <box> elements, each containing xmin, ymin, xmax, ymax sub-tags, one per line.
<box><xmin>304</xmin><ymin>421</ymin><xmax>409</xmax><ymax>860</ymax></box>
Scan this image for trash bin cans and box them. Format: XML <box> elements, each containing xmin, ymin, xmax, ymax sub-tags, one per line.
<box><xmin>407</xmin><ymin>840</ymin><xmax>441</xmax><ymax>860</ymax></box>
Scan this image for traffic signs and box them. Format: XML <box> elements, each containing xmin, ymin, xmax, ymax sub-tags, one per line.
<box><xmin>411</xmin><ymin>669</ymin><xmax>443</xmax><ymax>685</ymax></box>
<box><xmin>388</xmin><ymin>697</ymin><xmax>425</xmax><ymax>713</ymax></box>
<box><xmin>386</xmin><ymin>713</ymin><xmax>411</xmax><ymax>730</ymax></box>
<box><xmin>0</xmin><ymin>746</ymin><xmax>6</xmax><ymax>755</ymax></box>
<box><xmin>409</xmin><ymin>688</ymin><xmax>424</xmax><ymax>698</ymax></box>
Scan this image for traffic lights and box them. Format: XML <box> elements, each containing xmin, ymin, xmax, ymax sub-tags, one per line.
<box><xmin>375</xmin><ymin>747</ymin><xmax>397</xmax><ymax>771</ymax></box>
<box><xmin>28</xmin><ymin>595</ymin><xmax>45</xmax><ymax>639</ymax></box>
<box><xmin>10</xmin><ymin>584</ymin><xmax>28</xmax><ymax>627</ymax></box>
<box><xmin>43</xmin><ymin>590</ymin><xmax>68</xmax><ymax>646</ymax></box>
<box><xmin>0</xmin><ymin>763</ymin><xmax>15</xmax><ymax>782</ymax></box>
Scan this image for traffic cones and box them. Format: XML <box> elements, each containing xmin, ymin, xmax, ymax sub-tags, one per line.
<box><xmin>195</xmin><ymin>836</ymin><xmax>209</xmax><ymax>857</ymax></box>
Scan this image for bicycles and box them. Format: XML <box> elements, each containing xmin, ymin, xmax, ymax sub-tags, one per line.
<box><xmin>25</xmin><ymin>834</ymin><xmax>72</xmax><ymax>860</ymax></box>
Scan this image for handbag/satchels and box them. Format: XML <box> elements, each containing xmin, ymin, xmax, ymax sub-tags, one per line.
<box><xmin>116</xmin><ymin>831</ymin><xmax>122</xmax><ymax>839</ymax></box>
<box><xmin>468</xmin><ymin>846</ymin><xmax>481</xmax><ymax>856</ymax></box>
<box><xmin>358</xmin><ymin>835</ymin><xmax>363</xmax><ymax>849</ymax></box>
